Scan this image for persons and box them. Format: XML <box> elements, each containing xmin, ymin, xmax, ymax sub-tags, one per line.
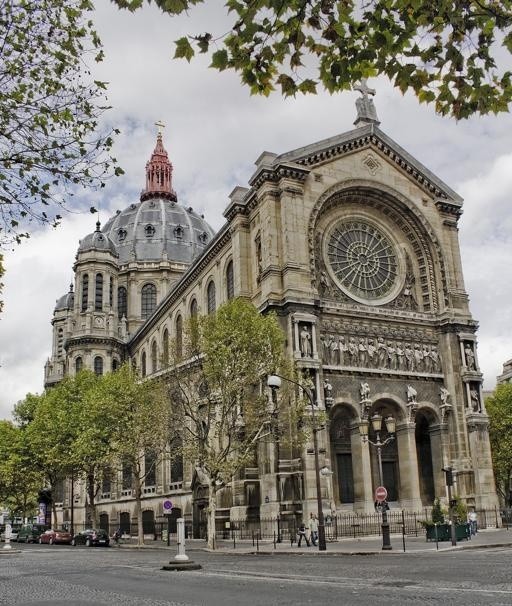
<box><xmin>405</xmin><ymin>383</ymin><xmax>417</xmax><ymax>403</ymax></box>
<box><xmin>375</xmin><ymin>498</ymin><xmax>390</xmax><ymax>511</ymax></box>
<box><xmin>302</xmin><ymin>369</ymin><xmax>315</xmax><ymax>404</ymax></box>
<box><xmin>358</xmin><ymin>380</ymin><xmax>370</xmax><ymax>400</ymax></box>
<box><xmin>470</xmin><ymin>384</ymin><xmax>482</xmax><ymax>413</ymax></box>
<box><xmin>439</xmin><ymin>385</ymin><xmax>450</xmax><ymax>405</ymax></box>
<box><xmin>307</xmin><ymin>514</ymin><xmax>319</xmax><ymax>546</ymax></box>
<box><xmin>113</xmin><ymin>531</ymin><xmax>118</xmax><ymax>544</ymax></box>
<box><xmin>467</xmin><ymin>508</ymin><xmax>478</xmax><ymax>537</ymax></box>
<box><xmin>319</xmin><ymin>332</ymin><xmax>443</xmax><ymax>374</ymax></box>
<box><xmin>464</xmin><ymin>342</ymin><xmax>476</xmax><ymax>372</ymax></box>
<box><xmin>322</xmin><ymin>378</ymin><xmax>333</xmax><ymax>399</ymax></box>
<box><xmin>298</xmin><ymin>518</ymin><xmax>311</xmax><ymax>547</ymax></box>
<box><xmin>300</xmin><ymin>325</ymin><xmax>312</xmax><ymax>358</ymax></box>
<box><xmin>320</xmin><ymin>271</ymin><xmax>331</xmax><ymax>298</ymax></box>
<box><xmin>403</xmin><ymin>284</ymin><xmax>413</xmax><ymax>311</ymax></box>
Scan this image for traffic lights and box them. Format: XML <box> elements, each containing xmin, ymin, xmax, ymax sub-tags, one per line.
<box><xmin>441</xmin><ymin>464</ymin><xmax>459</xmax><ymax>486</ymax></box>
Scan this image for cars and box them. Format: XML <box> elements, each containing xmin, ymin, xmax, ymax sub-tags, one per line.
<box><xmin>0</xmin><ymin>524</ymin><xmax>109</xmax><ymax>547</ymax></box>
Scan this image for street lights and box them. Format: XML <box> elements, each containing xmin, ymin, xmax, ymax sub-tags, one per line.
<box><xmin>358</xmin><ymin>411</ymin><xmax>397</xmax><ymax>550</ymax></box>
<box><xmin>267</xmin><ymin>373</ymin><xmax>325</xmax><ymax>551</ymax></box>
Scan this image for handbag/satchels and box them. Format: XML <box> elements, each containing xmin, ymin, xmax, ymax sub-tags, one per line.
<box><xmin>297</xmin><ymin>527</ymin><xmax>306</xmax><ymax>535</ymax></box>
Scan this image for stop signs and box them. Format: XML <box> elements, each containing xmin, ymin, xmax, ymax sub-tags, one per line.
<box><xmin>375</xmin><ymin>487</ymin><xmax>388</xmax><ymax>502</ymax></box>
